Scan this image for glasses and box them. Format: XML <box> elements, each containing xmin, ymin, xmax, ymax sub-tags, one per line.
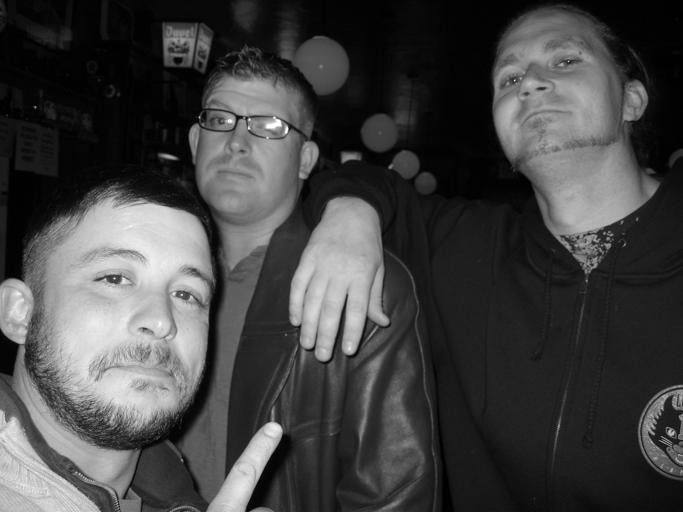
<box><xmin>198</xmin><ymin>108</ymin><xmax>308</xmax><ymax>139</ymax></box>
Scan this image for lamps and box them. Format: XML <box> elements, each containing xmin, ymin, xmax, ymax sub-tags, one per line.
<box><xmin>161</xmin><ymin>19</ymin><xmax>215</xmax><ymax>85</ymax></box>
<box><xmin>292</xmin><ymin>34</ymin><xmax>352</xmax><ymax>96</ymax></box>
<box><xmin>358</xmin><ymin>65</ymin><xmax>439</xmax><ymax>196</ymax></box>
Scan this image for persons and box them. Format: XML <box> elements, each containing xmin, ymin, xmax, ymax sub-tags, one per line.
<box><xmin>288</xmin><ymin>0</ymin><xmax>681</xmax><ymax>510</ymax></box>
<box><xmin>166</xmin><ymin>47</ymin><xmax>443</xmax><ymax>511</ymax></box>
<box><xmin>0</xmin><ymin>160</ymin><xmax>285</xmax><ymax>512</ymax></box>
